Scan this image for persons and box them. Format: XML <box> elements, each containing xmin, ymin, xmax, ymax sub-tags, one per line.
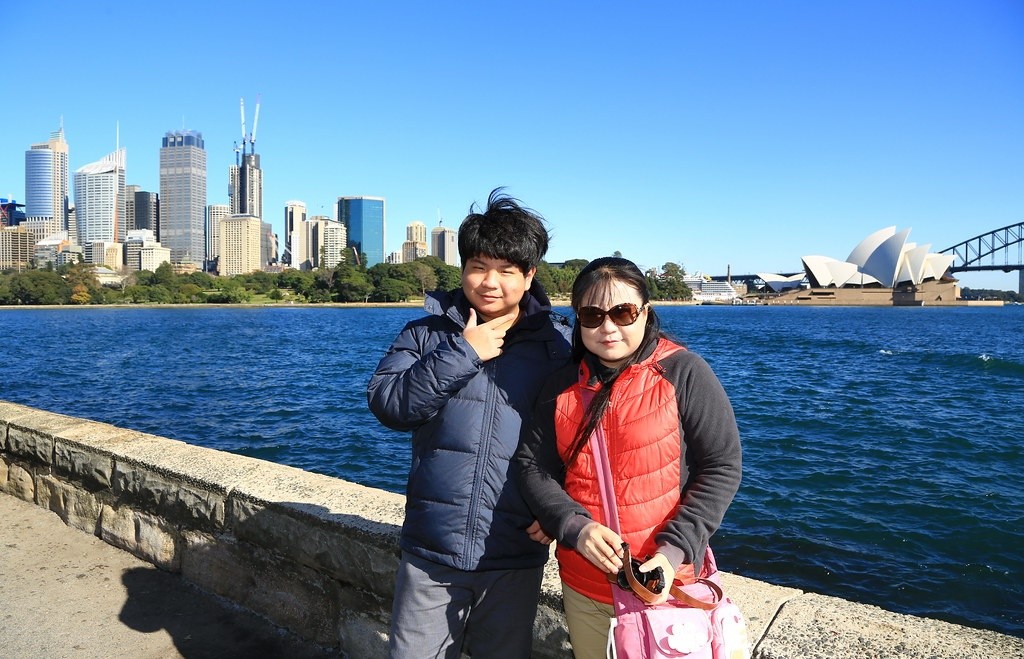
<box><xmin>513</xmin><ymin>256</ymin><xmax>743</xmax><ymax>659</ymax></box>
<box><xmin>366</xmin><ymin>188</ymin><xmax>572</xmax><ymax>659</ymax></box>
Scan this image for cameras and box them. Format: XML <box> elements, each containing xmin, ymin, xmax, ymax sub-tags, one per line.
<box><xmin>618</xmin><ymin>558</ymin><xmax>665</xmax><ymax>594</ymax></box>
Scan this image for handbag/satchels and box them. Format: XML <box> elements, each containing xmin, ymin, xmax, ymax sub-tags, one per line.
<box><xmin>606</xmin><ymin>570</ymin><xmax>750</xmax><ymax>659</ymax></box>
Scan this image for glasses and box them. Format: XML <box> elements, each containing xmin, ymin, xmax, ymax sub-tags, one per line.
<box><xmin>577</xmin><ymin>303</ymin><xmax>645</xmax><ymax>329</ymax></box>
